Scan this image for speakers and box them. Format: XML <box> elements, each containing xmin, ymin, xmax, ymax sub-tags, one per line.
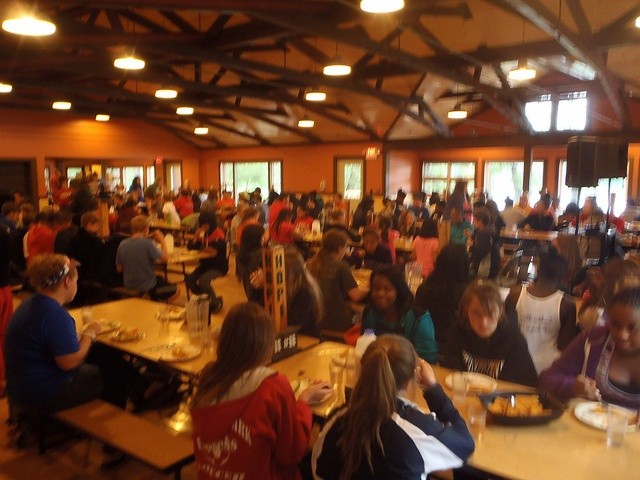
<box><xmin>599</xmin><ymin>134</ymin><xmax>631</xmax><ymax>180</ymax></box>
<box><xmin>564</xmin><ymin>136</ymin><xmax>599</xmax><ymax>188</ymax></box>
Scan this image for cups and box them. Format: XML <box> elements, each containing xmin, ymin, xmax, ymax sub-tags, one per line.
<box><xmin>345</xmin><ymin>353</ymin><xmax>358</xmax><ymax>388</ymax></box>
<box><xmin>184</xmin><ymin>299</ymin><xmax>209</xmax><ymax>336</ymax></box>
<box><xmin>450</xmin><ymin>369</ymin><xmax>469</xmax><ymax>403</ymax></box>
<box><xmin>198</xmin><ymin>325</ymin><xmax>217</xmax><ymax>353</ymax></box>
<box><xmin>158</xmin><ymin>306</ymin><xmax>170</xmax><ymax>328</ymax></box>
<box><xmin>329</xmin><ymin>360</ymin><xmax>345</xmax><ymax>398</ymax></box>
<box><xmin>81</xmin><ymin>307</ymin><xmax>94</xmax><ymax>325</ymax></box>
<box><xmin>603</xmin><ymin>415</ymin><xmax>627</xmax><ymax>449</ymax></box>
<box><xmin>402</xmin><ymin>261</ymin><xmax>422</xmax><ymax>288</ymax></box>
<box><xmin>468</xmin><ymin>405</ymin><xmax>487</xmax><ymax>436</ymax></box>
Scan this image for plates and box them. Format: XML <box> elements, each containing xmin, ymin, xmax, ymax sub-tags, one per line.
<box><xmin>575</xmin><ymin>400</ymin><xmax>640</xmax><ymax>433</ymax></box>
<box><xmin>444</xmin><ymin>370</ymin><xmax>499</xmax><ymax>396</ymax></box>
<box><xmin>84</xmin><ymin>317</ymin><xmax>123</xmax><ymax>335</ymax></box>
<box><xmin>478</xmin><ymin>391</ymin><xmax>565</xmax><ymax>426</ymax></box>
<box><xmin>161</xmin><ymin>344</ymin><xmax>202</xmax><ymax>363</ymax></box>
<box><xmin>291</xmin><ymin>378</ymin><xmax>334</xmax><ymax>404</ymax></box>
<box><xmin>109</xmin><ymin>327</ymin><xmax>147</xmax><ymax>342</ymax></box>
<box><xmin>332</xmin><ymin>348</ymin><xmax>357</xmax><ymax>369</ymax></box>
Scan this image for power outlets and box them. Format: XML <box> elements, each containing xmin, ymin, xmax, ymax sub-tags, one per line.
<box><xmin>306</xmin><ymin>41</ymin><xmax>326</xmax><ymax>103</ymax></box>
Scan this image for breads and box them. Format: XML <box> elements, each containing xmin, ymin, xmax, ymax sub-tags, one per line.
<box><xmin>490</xmin><ymin>393</ymin><xmax>545</xmax><ymax>421</ymax></box>
<box><xmin>111</xmin><ymin>325</ymin><xmax>143</xmax><ymax>342</ymax></box>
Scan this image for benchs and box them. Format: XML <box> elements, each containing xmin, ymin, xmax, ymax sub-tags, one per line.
<box><xmin>54</xmin><ymin>401</ymin><xmax>195</xmax><ymax>480</ymax></box>
<box><xmin>155</xmin><ymin>264</ymin><xmax>199</xmax><ymax>276</ymax></box>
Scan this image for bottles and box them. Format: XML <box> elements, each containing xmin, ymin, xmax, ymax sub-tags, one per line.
<box><xmin>164</xmin><ymin>233</ymin><xmax>174</xmax><ymax>257</ymax></box>
<box><xmin>354</xmin><ymin>328</ymin><xmax>378</xmax><ymax>373</ymax></box>
<box><xmin>310</xmin><ymin>219</ymin><xmax>321</xmax><ymax>236</ymax></box>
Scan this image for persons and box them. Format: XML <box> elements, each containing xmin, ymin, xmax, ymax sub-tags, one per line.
<box><xmin>463</xmin><ymin>191</ymin><xmax>505</xmax><ymax>277</ymax></box>
<box><xmin>69</xmin><ymin>170</ymin><xmax>194</xmax><ymax>231</ymax></box>
<box><xmin>66</xmin><ymin>210</ymin><xmax>107</xmax><ymax>288</ymax></box>
<box><xmin>246</xmin><ymin>186</ymin><xmax>262</xmax><ymax>207</ymax></box>
<box><xmin>188</xmin><ymin>301</ymin><xmax>331</xmax><ymax>479</ymax></box>
<box><xmin>442</xmin><ymin>278</ymin><xmax>538</xmax><ymax>389</ymax></box>
<box><xmin>194</xmin><ymin>185</ymin><xmax>235</xmax><ymax>228</ymax></box>
<box><xmin>506</xmin><ymin>252</ymin><xmax>577</xmax><ymax>375</ymax></box>
<box><xmin>265</xmin><ymin>185</ymin><xmax>323</xmax><ymax>246</ymax></box>
<box><xmin>323</xmin><ymin>193</ymin><xmax>359</xmax><ymax>242</ymax></box>
<box><xmin>185</xmin><ymin>211</ymin><xmax>229</xmax><ymax>313</ymax></box>
<box><xmin>310</xmin><ymin>333</ymin><xmax>475</xmax><ymax>480</ymax></box>
<box><xmin>407</xmin><ymin>192</ymin><xmax>441</xmax><ymax>279</ymax></box>
<box><xmin>414</xmin><ymin>243</ymin><xmax>476</xmax><ymax>355</ymax></box>
<box><xmin>441</xmin><ymin>177</ymin><xmax>472</xmax><ymax>243</ymax></box>
<box><xmin>253</xmin><ymin>195</ymin><xmax>266</xmax><ymax>225</ymax></box>
<box><xmin>579</xmin><ymin>261</ymin><xmax>640</xmax><ymax>329</ymax></box>
<box><xmin>236</xmin><ymin>192</ymin><xmax>247</xmax><ymax>208</ymax></box>
<box><xmin>248</xmin><ymin>253</ymin><xmax>325</xmax><ymax>336</ymax></box>
<box><xmin>230</xmin><ymin>203</ymin><xmax>247</xmax><ymax>250</ymax></box>
<box><xmin>538</xmin><ymin>287</ymin><xmax>640</xmax><ymax>424</ymax></box>
<box><xmin>234</xmin><ymin>225</ymin><xmax>267</xmax><ymax>305</ymax></box>
<box><xmin>0</xmin><ymin>169</ymin><xmax>73</xmax><ymax>258</ymax></box>
<box><xmin>236</xmin><ymin>208</ymin><xmax>260</xmax><ymax>250</ymax></box>
<box><xmin>349</xmin><ymin>188</ymin><xmax>407</xmax><ymax>269</ymax></box>
<box><xmin>116</xmin><ymin>215</ymin><xmax>181</xmax><ymax>305</ymax></box>
<box><xmin>4</xmin><ymin>253</ymin><xmax>128</xmax><ymax>455</ymax></box>
<box><xmin>502</xmin><ymin>186</ymin><xmax>640</xmax><ymax>241</ymax></box>
<box><xmin>359</xmin><ymin>263</ymin><xmax>439</xmax><ymax>365</ymax></box>
<box><xmin>304</xmin><ymin>228</ymin><xmax>370</xmax><ymax>332</ymax></box>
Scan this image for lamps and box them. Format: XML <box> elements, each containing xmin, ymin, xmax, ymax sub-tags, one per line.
<box><xmin>297</xmin><ymin>106</ymin><xmax>315</xmax><ymax>129</ymax></box>
<box><xmin>322</xmin><ymin>38</ymin><xmax>352</xmax><ymax>76</ymax></box>
<box><xmin>446</xmin><ymin>81</ymin><xmax>468</xmax><ymax>119</ymax></box>
<box><xmin>195</xmin><ymin>118</ymin><xmax>208</xmax><ymax>136</ymax></box>
<box><xmin>508</xmin><ymin>17</ymin><xmax>536</xmax><ymax>83</ymax></box>
<box><xmin>114</xmin><ymin>12</ymin><xmax>146</xmax><ymax>71</ymax></box>
<box><xmin>155</xmin><ymin>67</ymin><xmax>178</xmax><ymax>100</ymax></box>
<box><xmin>176</xmin><ymin>90</ymin><xmax>194</xmax><ymax>116</ymax></box>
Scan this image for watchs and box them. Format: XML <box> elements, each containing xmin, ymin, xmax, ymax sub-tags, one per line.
<box><xmin>83</xmin><ymin>332</ymin><xmax>98</xmax><ymax>343</ymax></box>
